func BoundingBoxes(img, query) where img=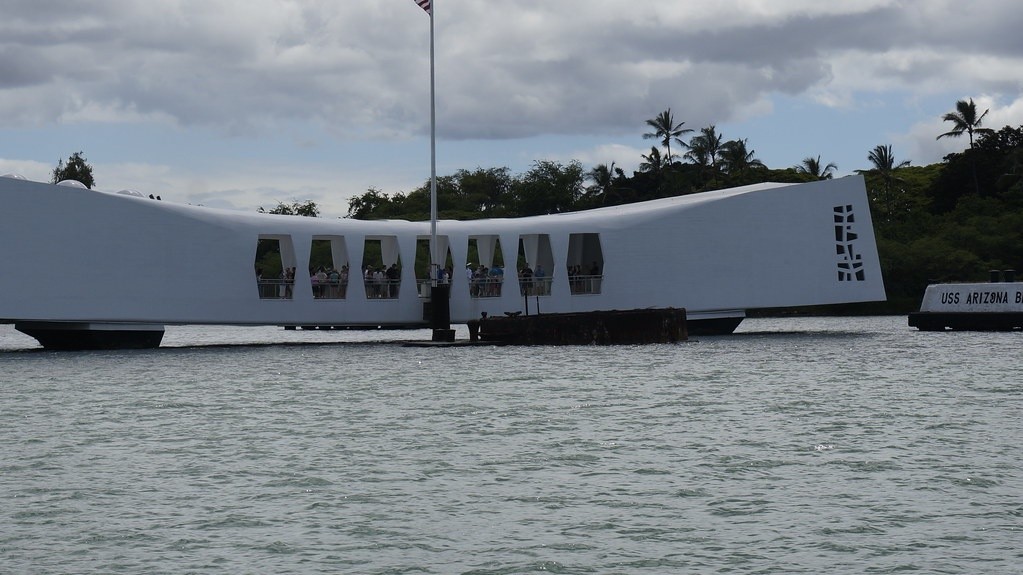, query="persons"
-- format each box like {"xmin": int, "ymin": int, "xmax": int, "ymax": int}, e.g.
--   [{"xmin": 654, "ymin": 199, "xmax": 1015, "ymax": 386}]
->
[{"xmin": 257, "ymin": 263, "xmax": 600, "ymax": 299}]
